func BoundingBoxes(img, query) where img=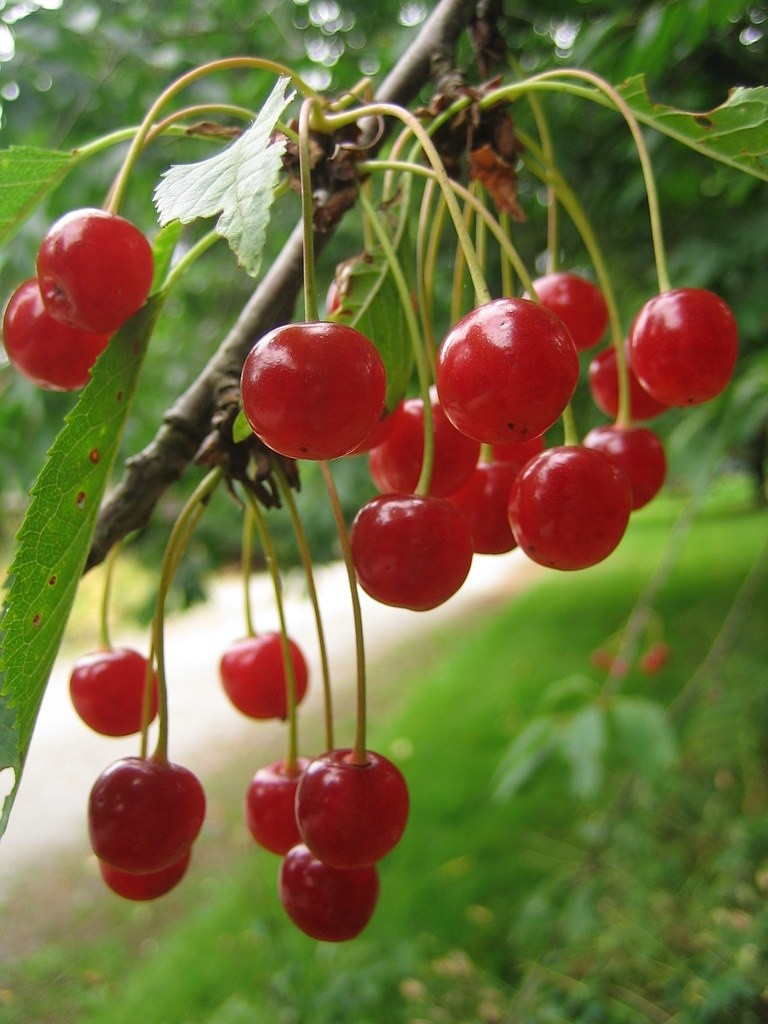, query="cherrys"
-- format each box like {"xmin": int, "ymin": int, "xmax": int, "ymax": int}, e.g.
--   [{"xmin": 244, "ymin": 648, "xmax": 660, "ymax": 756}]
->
[{"xmin": 1, "ymin": 60, "xmax": 738, "ymax": 942}]
